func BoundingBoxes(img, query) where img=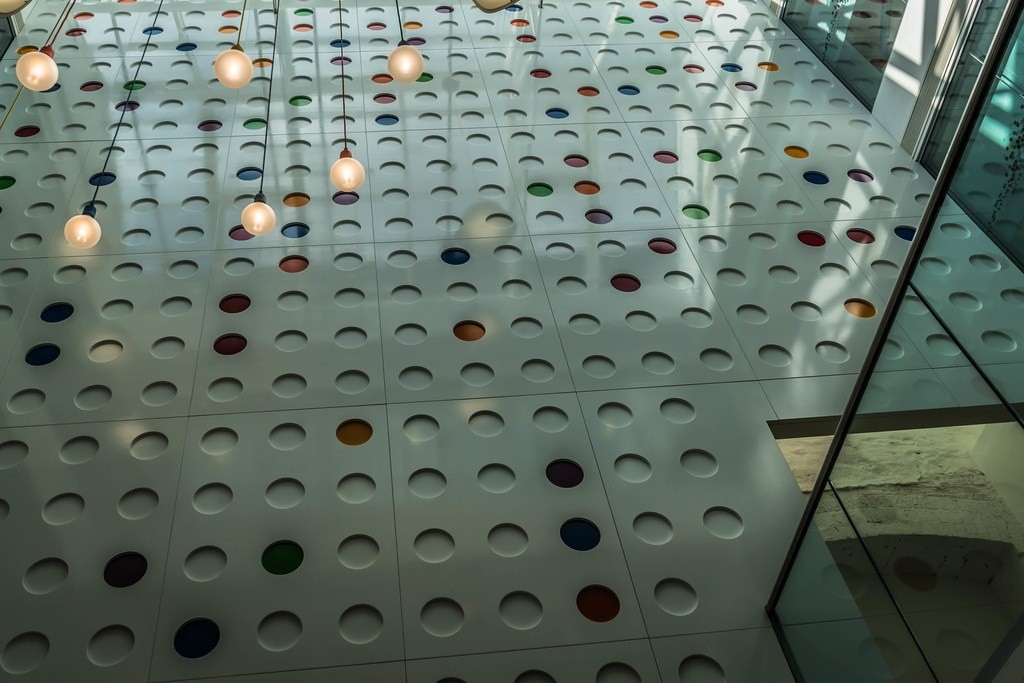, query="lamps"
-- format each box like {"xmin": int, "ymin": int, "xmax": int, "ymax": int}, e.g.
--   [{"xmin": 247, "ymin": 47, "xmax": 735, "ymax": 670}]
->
[
  {"xmin": 63, "ymin": 1, "xmax": 165, "ymax": 250},
  {"xmin": 214, "ymin": 0, "xmax": 255, "ymax": 89},
  {"xmin": 386, "ymin": 0, "xmax": 425, "ymax": 84},
  {"xmin": 240, "ymin": 0, "xmax": 280, "ymax": 236},
  {"xmin": 14, "ymin": 0, "xmax": 77, "ymax": 92},
  {"xmin": 328, "ymin": 0, "xmax": 365, "ymax": 192}
]
